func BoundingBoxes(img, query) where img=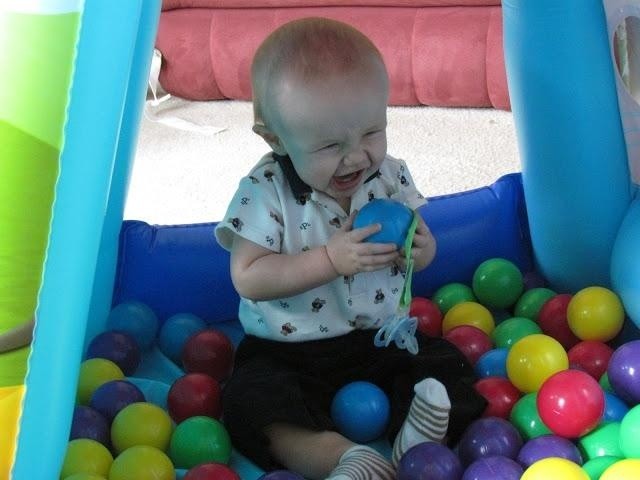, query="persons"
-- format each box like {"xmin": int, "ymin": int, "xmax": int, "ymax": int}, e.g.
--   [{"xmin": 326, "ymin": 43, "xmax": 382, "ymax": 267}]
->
[{"xmin": 212, "ymin": 16, "xmax": 489, "ymax": 480}]
